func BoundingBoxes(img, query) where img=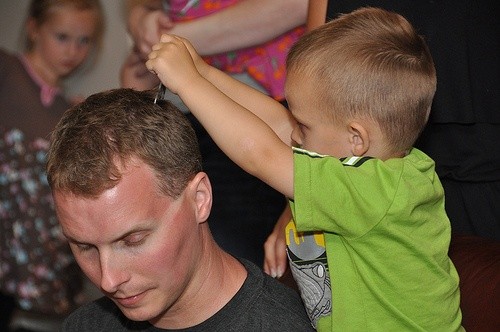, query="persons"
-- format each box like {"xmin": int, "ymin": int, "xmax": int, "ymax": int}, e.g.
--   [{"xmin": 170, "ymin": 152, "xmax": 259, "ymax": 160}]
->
[
  {"xmin": 256, "ymin": 1, "xmax": 499, "ymax": 280},
  {"xmin": 121, "ymin": 1, "xmax": 329, "ymax": 268},
  {"xmin": 143, "ymin": 6, "xmax": 470, "ymax": 332},
  {"xmin": 0, "ymin": 0, "xmax": 108, "ymax": 330},
  {"xmin": 43, "ymin": 89, "xmax": 315, "ymax": 332}
]
